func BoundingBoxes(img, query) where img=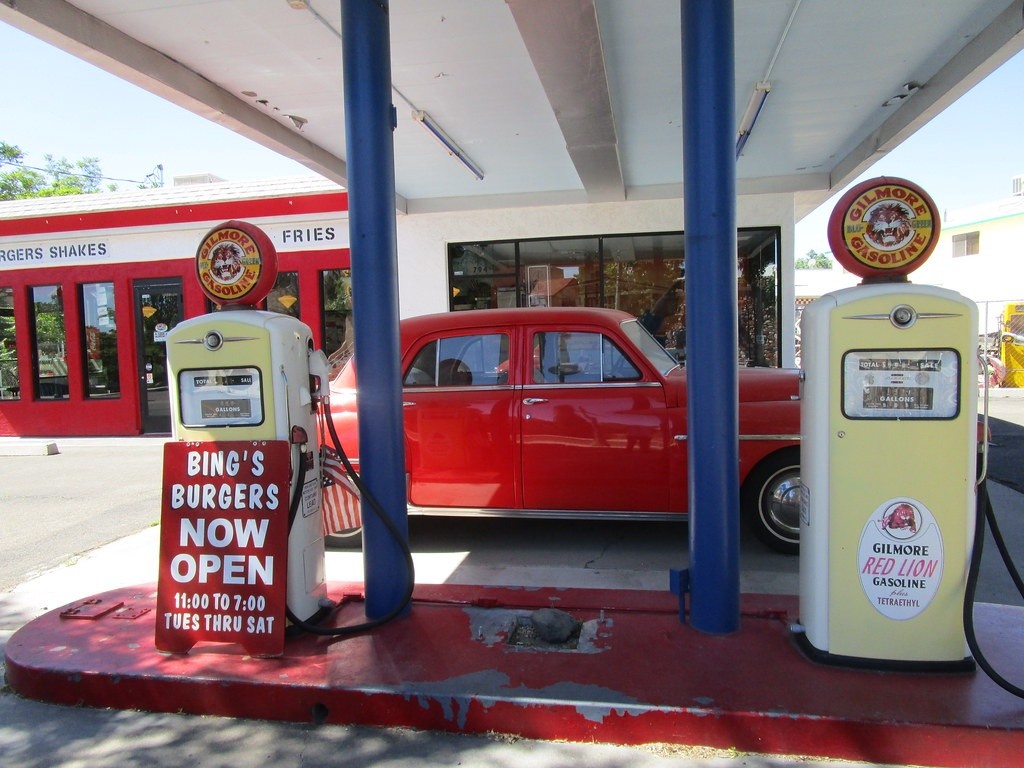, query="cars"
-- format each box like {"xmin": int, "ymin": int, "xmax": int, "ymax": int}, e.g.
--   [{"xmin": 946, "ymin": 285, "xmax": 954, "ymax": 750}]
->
[
  {"xmin": 494, "ymin": 338, "xmax": 637, "ymax": 378},
  {"xmin": 316, "ymin": 308, "xmax": 992, "ymax": 554}
]
